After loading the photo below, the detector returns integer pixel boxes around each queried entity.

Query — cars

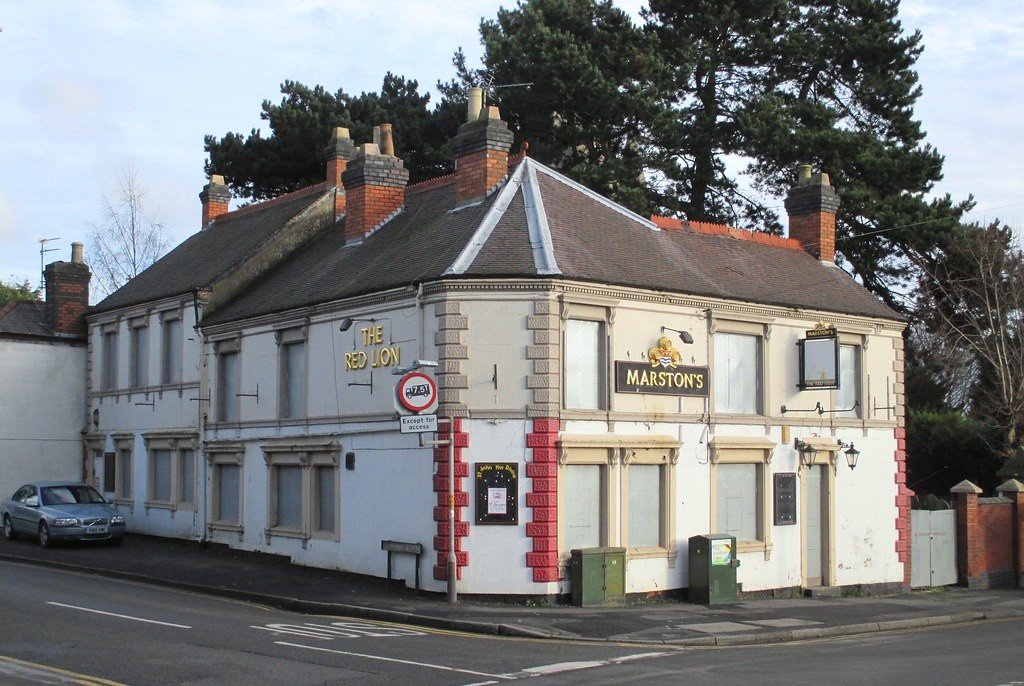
[0,479,127,549]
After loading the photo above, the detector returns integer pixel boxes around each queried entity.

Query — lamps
[838,439,860,471]
[795,438,817,470]
[662,326,694,344]
[340,317,375,332]
